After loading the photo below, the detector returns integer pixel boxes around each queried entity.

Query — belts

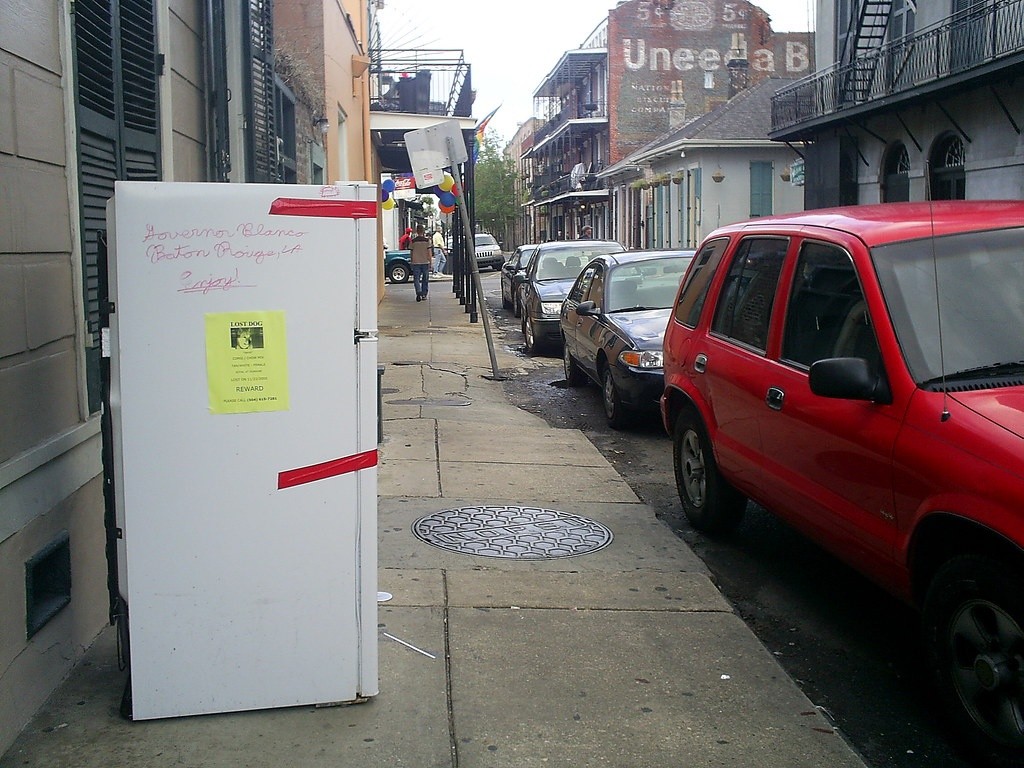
[434,247,440,248]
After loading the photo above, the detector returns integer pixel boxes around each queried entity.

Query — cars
[383,243,412,284]
[514,241,629,351]
[500,244,540,317]
[559,250,698,428]
[460,233,505,270]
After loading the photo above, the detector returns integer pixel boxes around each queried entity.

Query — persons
[399,228,412,250]
[433,226,447,278]
[579,225,594,239]
[411,224,432,302]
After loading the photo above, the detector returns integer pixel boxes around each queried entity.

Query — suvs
[660,198,1024,768]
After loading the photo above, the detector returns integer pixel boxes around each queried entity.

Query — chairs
[539,256,558,277]
[566,255,582,276]
[610,280,641,309]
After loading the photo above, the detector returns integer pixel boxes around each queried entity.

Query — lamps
[312,117,330,134]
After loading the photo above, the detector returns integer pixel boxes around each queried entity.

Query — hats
[435,226,442,231]
[405,228,412,232]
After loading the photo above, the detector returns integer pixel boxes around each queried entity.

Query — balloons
[382,190,389,202]
[382,198,394,210]
[434,175,459,213]
[383,179,395,192]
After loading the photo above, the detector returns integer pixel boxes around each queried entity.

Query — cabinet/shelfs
[399,70,432,115]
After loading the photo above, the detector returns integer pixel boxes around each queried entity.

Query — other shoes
[433,273,438,278]
[422,294,426,300]
[416,296,421,302]
[438,272,444,276]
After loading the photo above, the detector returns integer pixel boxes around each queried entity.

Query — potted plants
[712,171,725,182]
[779,169,790,181]
[630,171,684,191]
